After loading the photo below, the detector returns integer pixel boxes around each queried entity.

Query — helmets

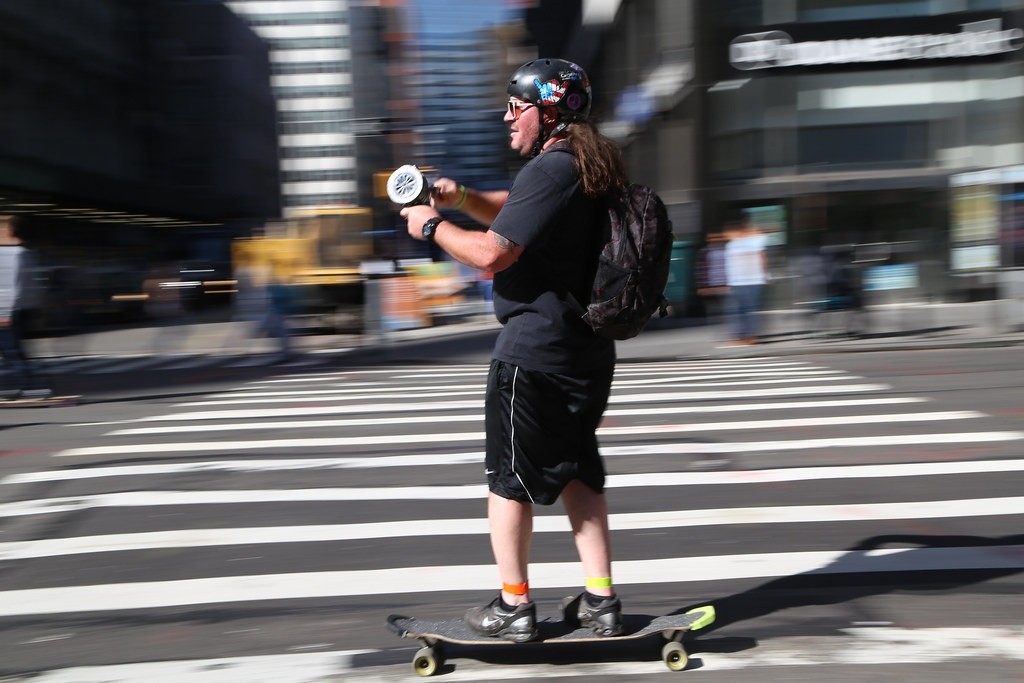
[506,58,592,124]
[548,121,548,124]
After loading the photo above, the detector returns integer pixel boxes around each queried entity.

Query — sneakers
[465,589,539,643]
[559,591,625,637]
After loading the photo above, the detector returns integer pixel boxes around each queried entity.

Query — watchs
[422,216,446,241]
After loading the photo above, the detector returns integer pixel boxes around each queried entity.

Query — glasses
[507,101,534,119]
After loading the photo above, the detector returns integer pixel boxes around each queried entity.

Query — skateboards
[382,604,716,678]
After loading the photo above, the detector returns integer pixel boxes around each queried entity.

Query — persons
[400,58,624,643]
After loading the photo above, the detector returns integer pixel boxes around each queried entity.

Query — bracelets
[452,184,467,210]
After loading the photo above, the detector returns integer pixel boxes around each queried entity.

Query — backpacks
[540,140,673,341]
